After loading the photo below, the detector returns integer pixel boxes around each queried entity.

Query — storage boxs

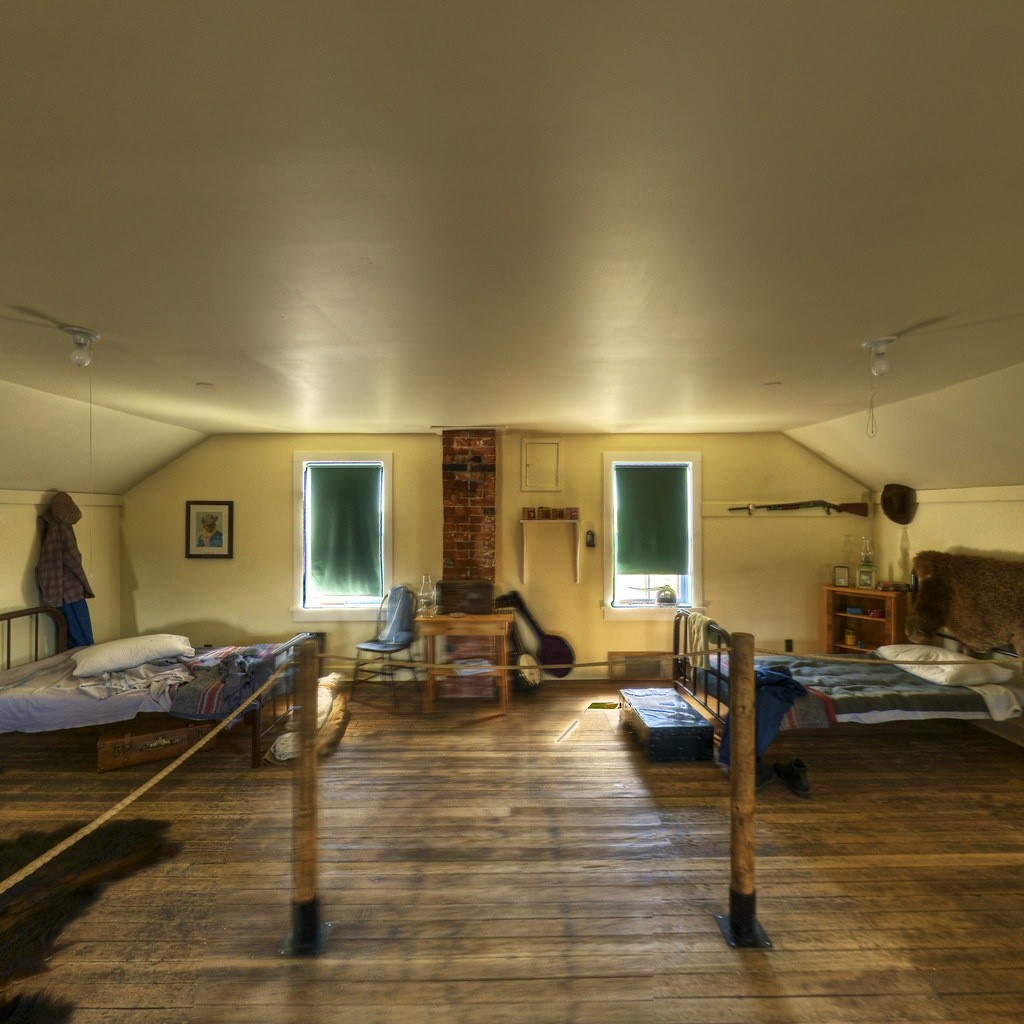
[436,581,497,614]
[619,687,714,759]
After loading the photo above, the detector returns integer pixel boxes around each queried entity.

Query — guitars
[502,592,578,688]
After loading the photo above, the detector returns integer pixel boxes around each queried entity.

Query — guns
[728,498,868,516]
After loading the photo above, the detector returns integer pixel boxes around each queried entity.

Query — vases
[859,535,878,564]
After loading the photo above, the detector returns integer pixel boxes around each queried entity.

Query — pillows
[71,632,195,678]
[877,644,1014,686]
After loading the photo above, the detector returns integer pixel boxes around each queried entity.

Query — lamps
[855,568,875,588]
[862,337,896,376]
[62,323,103,367]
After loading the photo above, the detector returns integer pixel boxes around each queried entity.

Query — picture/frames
[418,573,434,618]
[521,437,565,492]
[834,564,848,587]
[185,500,233,559]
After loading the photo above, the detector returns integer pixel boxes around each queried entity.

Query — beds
[0,607,320,769]
[671,550,1023,763]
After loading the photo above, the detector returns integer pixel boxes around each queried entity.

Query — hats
[201,514,219,524]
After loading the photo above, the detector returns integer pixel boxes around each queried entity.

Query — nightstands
[824,582,908,653]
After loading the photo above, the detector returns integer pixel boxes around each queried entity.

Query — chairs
[349,584,417,709]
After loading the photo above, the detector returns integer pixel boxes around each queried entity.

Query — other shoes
[773,759,810,797]
[756,756,776,793]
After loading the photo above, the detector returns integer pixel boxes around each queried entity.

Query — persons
[197,514,222,547]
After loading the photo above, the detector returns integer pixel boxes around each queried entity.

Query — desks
[421,611,514,716]
